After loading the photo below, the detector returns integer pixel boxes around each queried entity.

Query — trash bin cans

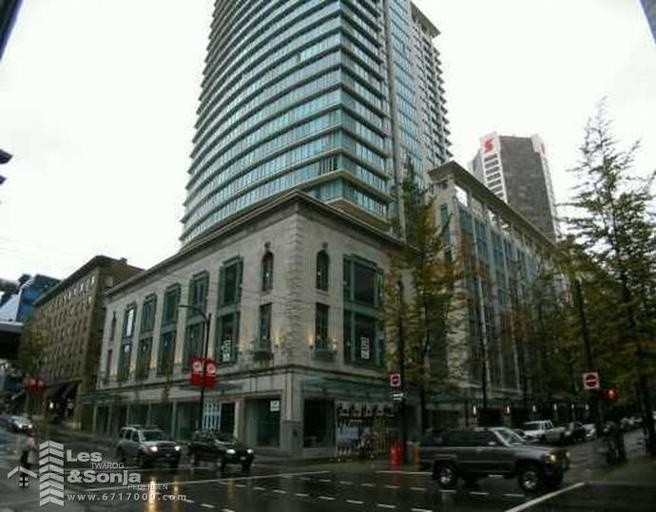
[596,441,614,469]
[391,442,401,465]
[407,441,420,463]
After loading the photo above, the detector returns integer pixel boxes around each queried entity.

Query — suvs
[114,423,182,470]
[417,425,572,495]
[187,428,256,471]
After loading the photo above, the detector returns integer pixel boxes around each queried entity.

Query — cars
[7,415,33,434]
[511,408,656,444]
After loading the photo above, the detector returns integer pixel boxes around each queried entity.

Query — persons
[20,437,37,489]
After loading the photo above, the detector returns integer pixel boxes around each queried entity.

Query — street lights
[175,303,212,429]
[463,398,590,425]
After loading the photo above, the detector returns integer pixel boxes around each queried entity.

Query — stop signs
[390,373,401,387]
[583,373,600,389]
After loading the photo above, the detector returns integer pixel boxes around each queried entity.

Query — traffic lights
[607,386,618,405]
